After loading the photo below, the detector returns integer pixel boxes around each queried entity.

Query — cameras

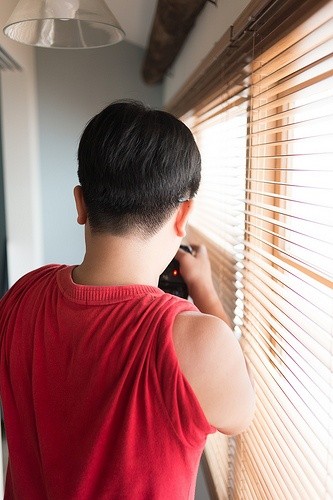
[159,245,191,300]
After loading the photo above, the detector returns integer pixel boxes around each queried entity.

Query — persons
[0,97,254,500]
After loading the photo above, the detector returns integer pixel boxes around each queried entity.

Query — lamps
[3,0,126,50]
